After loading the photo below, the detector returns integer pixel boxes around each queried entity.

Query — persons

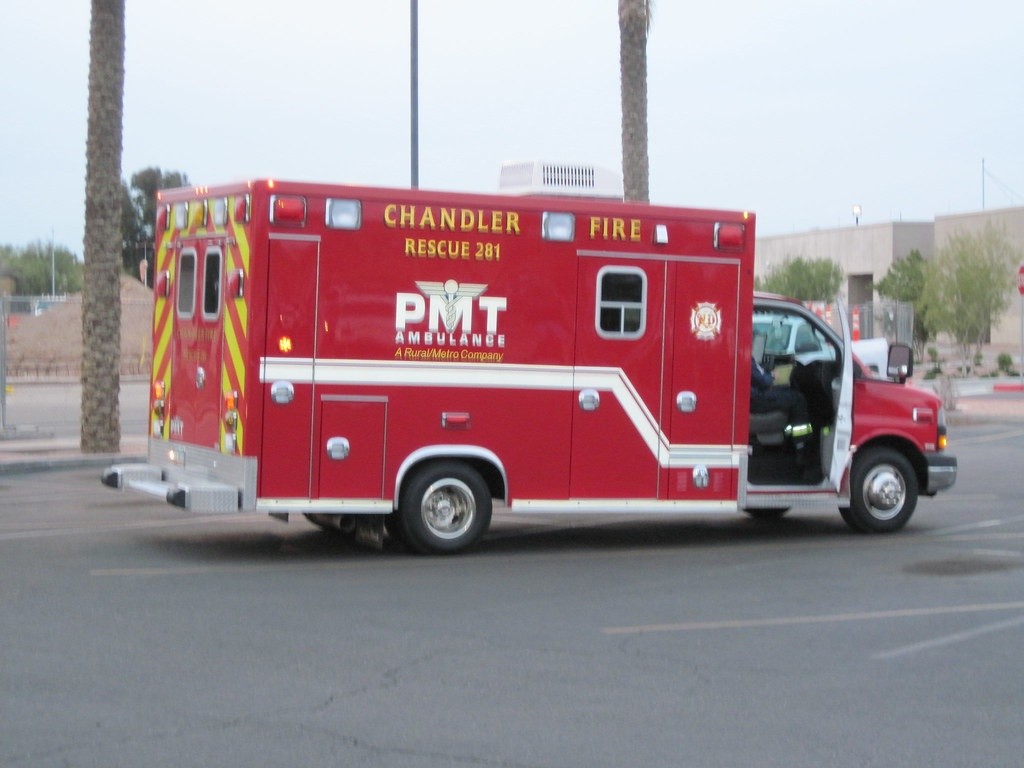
[750,355,815,466]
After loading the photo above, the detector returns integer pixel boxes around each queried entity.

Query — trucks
[752,312,898,387]
[99,158,958,560]
